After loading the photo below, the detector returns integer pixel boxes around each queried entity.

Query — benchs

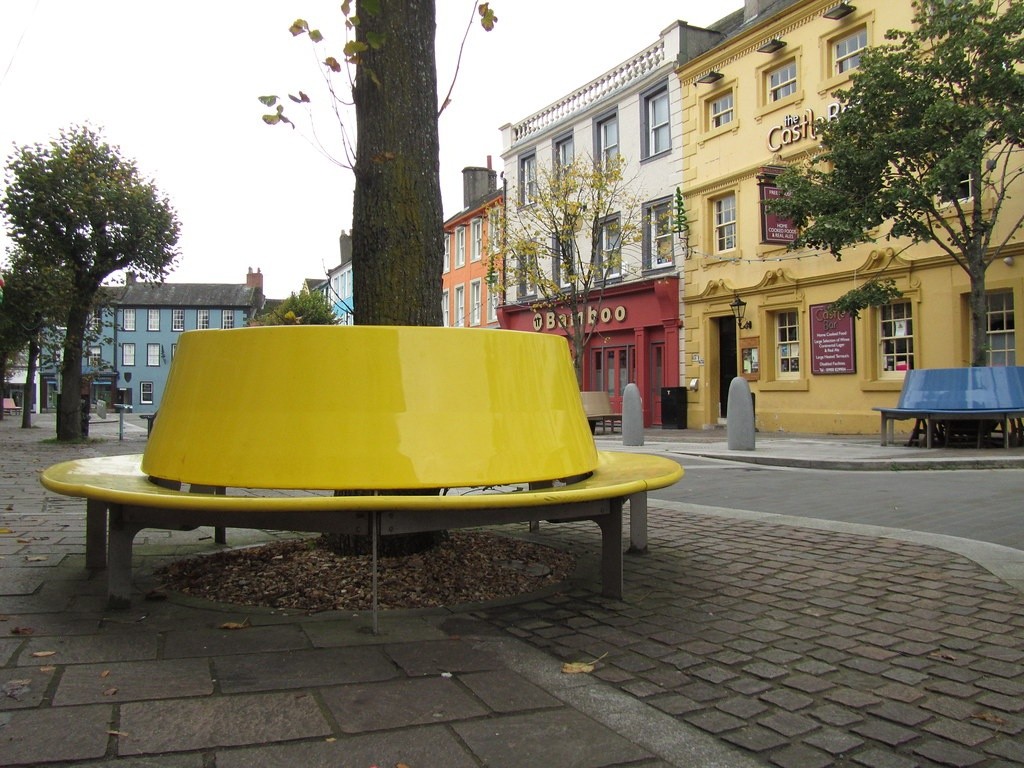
[41,454,686,602]
[3,398,22,416]
[872,366,1024,449]
[580,391,622,434]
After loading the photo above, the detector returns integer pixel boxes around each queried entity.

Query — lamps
[823,3,856,21]
[697,71,724,83]
[729,295,752,330]
[757,38,787,53]
